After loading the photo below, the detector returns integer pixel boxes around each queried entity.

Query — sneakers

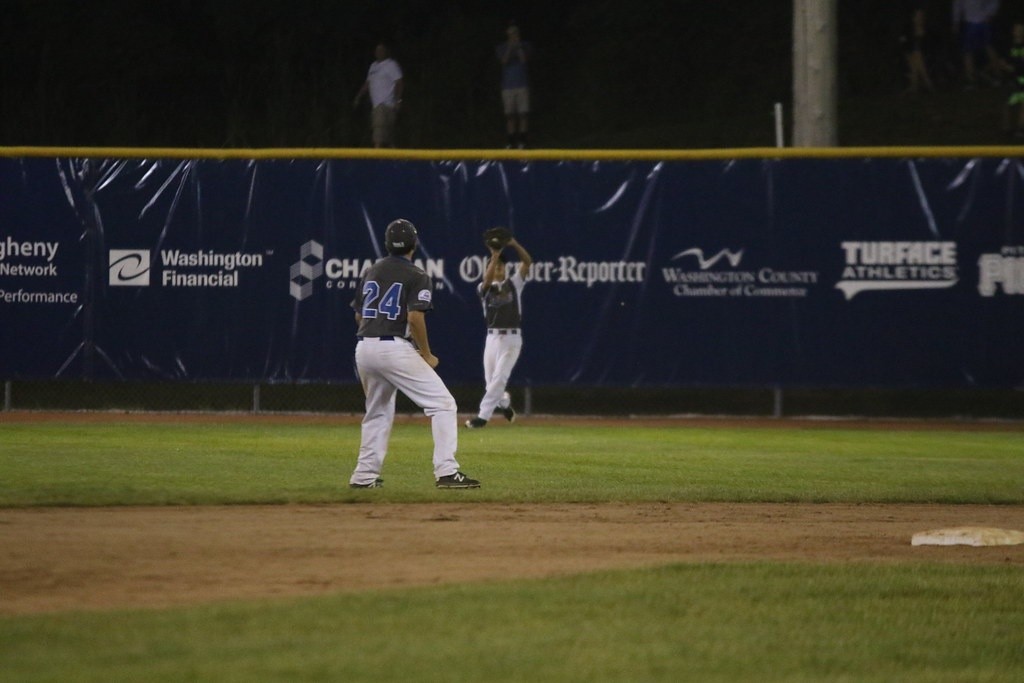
[436,472,480,489]
[349,478,384,489]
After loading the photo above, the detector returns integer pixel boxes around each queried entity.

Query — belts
[357,336,394,341]
[488,330,517,334]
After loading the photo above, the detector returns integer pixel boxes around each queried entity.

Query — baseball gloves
[484,226,512,250]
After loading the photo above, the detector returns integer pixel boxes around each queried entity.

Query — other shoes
[503,405,515,423]
[465,418,486,428]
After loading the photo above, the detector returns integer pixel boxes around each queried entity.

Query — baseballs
[491,237,499,242]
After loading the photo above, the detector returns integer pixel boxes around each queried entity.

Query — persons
[353,44,405,149]
[349,219,481,489]
[496,25,529,149]
[901,0,1024,137]
[465,227,532,429]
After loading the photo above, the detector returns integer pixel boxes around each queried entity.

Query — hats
[385,219,417,248]
[486,254,508,267]
